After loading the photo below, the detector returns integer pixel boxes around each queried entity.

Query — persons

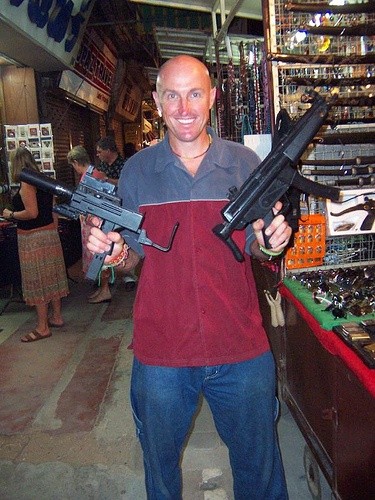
[67,144,115,304]
[93,136,126,189]
[123,142,138,158]
[88,54,292,500]
[3,147,71,343]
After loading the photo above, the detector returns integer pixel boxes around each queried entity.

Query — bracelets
[105,175,109,182]
[10,212,15,219]
[258,243,284,256]
[98,244,130,287]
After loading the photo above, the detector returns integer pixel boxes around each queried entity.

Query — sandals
[88,289,112,304]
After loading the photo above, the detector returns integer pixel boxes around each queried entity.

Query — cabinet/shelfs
[251,0,375,500]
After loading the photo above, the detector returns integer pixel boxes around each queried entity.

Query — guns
[211,89,341,263]
[18,164,180,283]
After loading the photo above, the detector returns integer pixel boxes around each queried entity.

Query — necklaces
[168,132,212,160]
[214,40,271,143]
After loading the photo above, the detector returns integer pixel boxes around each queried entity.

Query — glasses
[96,150,107,154]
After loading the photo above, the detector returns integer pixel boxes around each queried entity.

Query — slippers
[46,319,63,327]
[20,329,52,342]
